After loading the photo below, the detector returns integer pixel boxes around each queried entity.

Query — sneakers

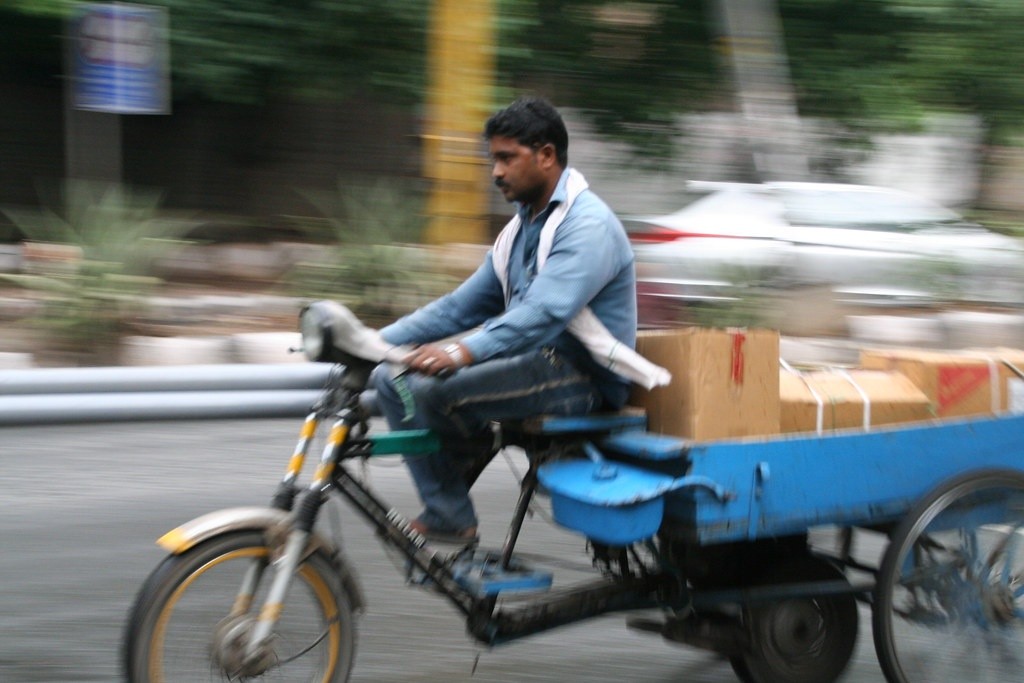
[406,517,479,544]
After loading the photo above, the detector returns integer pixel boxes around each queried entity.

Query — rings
[421,357,435,368]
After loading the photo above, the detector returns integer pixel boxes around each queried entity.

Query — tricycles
[120,299,1024,683]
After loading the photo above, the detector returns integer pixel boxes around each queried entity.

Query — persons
[373,95,640,546]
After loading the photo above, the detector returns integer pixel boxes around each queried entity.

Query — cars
[620,179,1024,349]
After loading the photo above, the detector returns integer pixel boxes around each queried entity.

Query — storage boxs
[856,345,1024,422]
[628,325,782,439]
[776,368,932,433]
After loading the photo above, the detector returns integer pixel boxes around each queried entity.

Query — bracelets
[442,343,466,367]
